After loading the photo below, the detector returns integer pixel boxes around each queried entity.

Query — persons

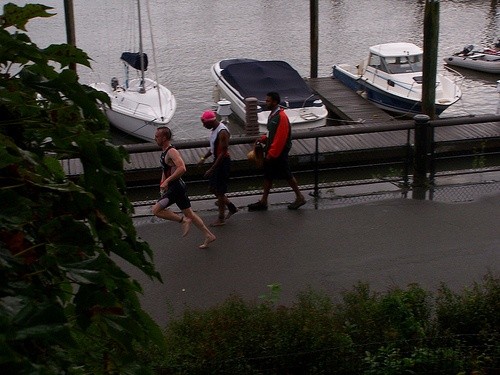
[248,91,307,210]
[152,127,216,249]
[196,110,240,227]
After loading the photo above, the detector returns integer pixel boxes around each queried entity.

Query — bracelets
[200,157,205,161]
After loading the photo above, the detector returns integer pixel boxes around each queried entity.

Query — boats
[210,58,328,134]
[443,45,500,74]
[87,0,177,143]
[330,42,465,117]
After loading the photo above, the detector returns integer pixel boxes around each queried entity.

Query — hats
[201,111,216,121]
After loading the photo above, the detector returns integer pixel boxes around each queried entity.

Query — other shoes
[288,200,306,209]
[249,202,267,211]
